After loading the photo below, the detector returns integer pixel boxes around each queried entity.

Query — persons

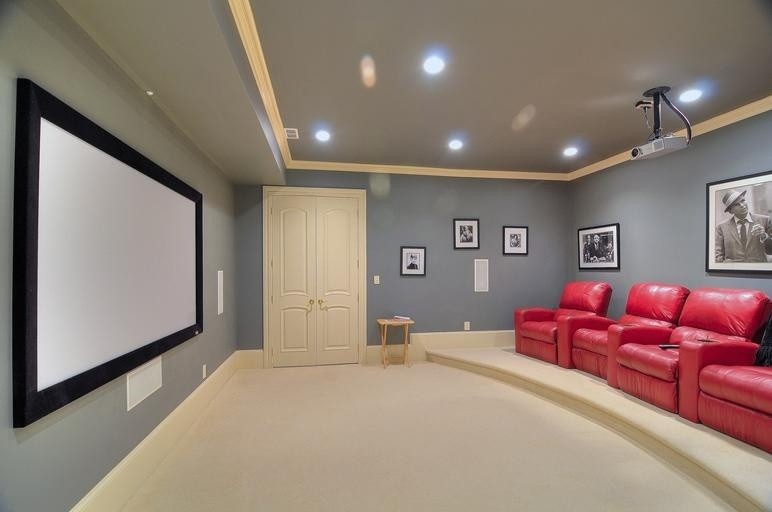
[460,225,473,242]
[715,189,772,263]
[406,253,419,269]
[511,235,519,248]
[583,234,613,262]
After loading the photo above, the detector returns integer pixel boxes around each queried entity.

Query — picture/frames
[453,218,480,249]
[578,223,620,270]
[400,245,426,276]
[503,226,528,255]
[706,169,772,275]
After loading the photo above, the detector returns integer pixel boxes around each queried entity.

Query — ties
[739,220,747,245]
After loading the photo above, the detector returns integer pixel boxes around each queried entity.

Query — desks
[377,319,415,369]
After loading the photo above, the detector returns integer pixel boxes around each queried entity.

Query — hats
[723,189,746,212]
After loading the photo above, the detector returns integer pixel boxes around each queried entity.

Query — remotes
[659,345,680,349]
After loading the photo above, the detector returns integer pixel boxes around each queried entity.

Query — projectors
[630,136,688,160]
